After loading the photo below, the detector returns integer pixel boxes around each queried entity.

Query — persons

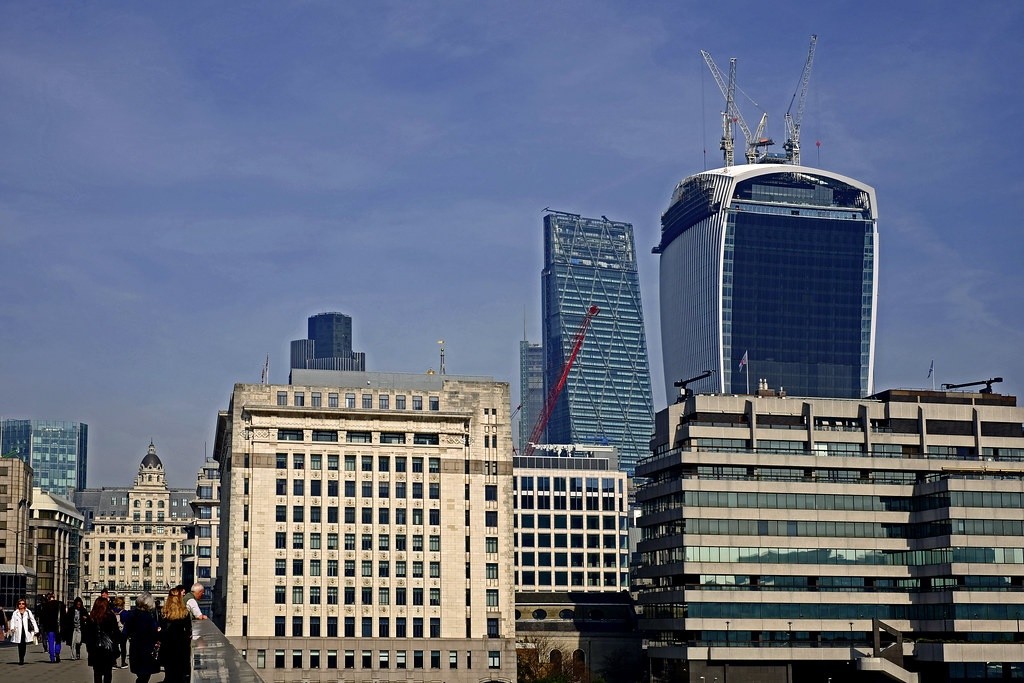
[83,597,122,683]
[66,597,90,660]
[101,588,128,670]
[124,591,161,683]
[157,583,207,683]
[36,592,66,663]
[10,599,39,665]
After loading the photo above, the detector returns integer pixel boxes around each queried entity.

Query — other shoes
[19,661,24,664]
[121,662,128,669]
[51,659,56,663]
[56,653,60,663]
[112,665,118,670]
[76,654,80,659]
[70,655,75,659]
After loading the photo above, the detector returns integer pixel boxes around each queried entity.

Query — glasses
[18,603,25,605]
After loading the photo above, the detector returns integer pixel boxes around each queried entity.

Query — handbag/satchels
[27,611,34,632]
[151,641,161,660]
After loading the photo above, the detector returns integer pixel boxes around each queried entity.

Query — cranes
[511,304,600,456]
[700,34,818,171]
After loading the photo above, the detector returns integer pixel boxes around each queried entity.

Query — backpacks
[112,609,125,632]
[94,616,119,653]
